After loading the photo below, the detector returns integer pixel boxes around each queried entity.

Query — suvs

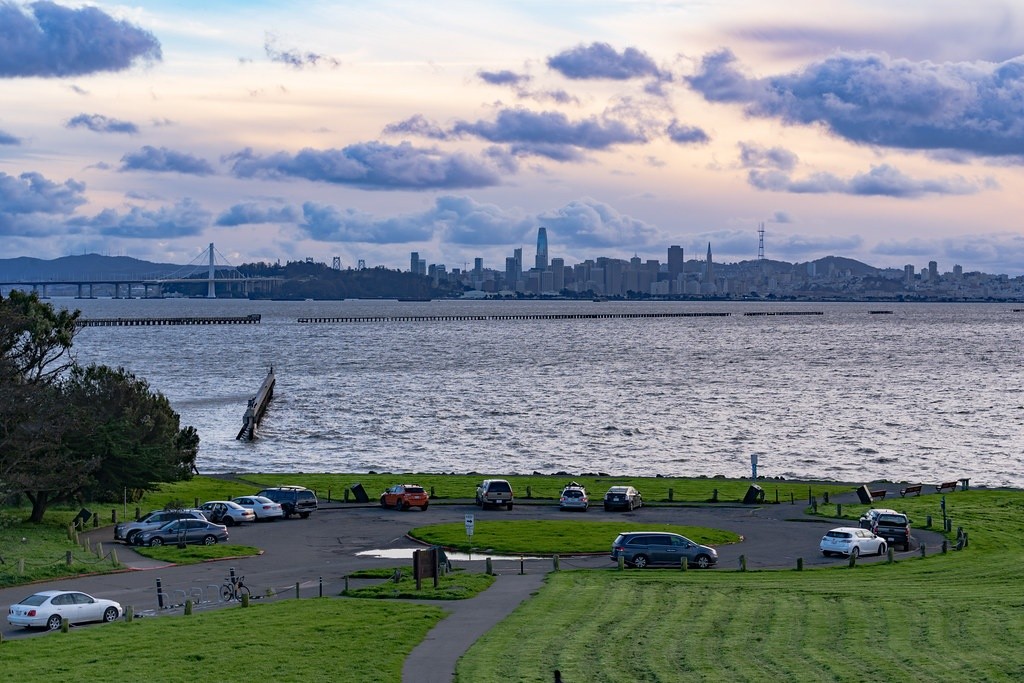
[558,485,591,512]
[256,486,318,519]
[475,480,515,510]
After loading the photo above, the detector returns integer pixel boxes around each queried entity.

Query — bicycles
[220,576,252,603]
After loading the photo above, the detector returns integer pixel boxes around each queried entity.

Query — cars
[6,589,123,630]
[232,496,285,522]
[112,509,229,548]
[611,532,719,568]
[821,527,886,558]
[378,484,431,513]
[605,486,644,512]
[197,501,256,525]
[859,507,914,549]
